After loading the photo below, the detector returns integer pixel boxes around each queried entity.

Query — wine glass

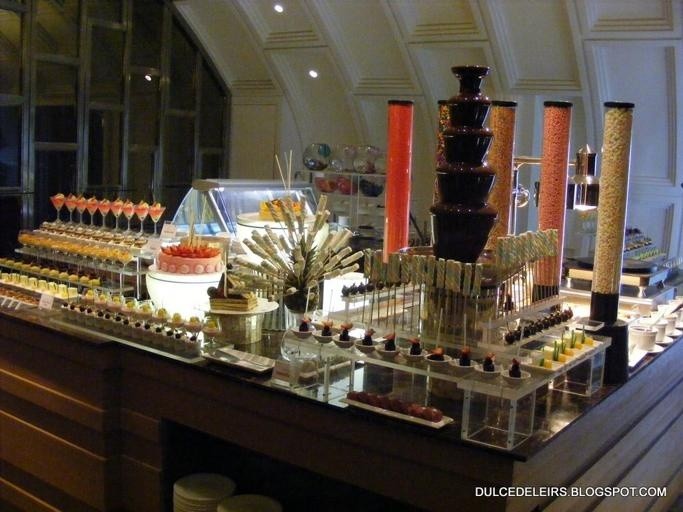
[64,200,77,228]
[121,207,136,234]
[148,206,167,237]
[50,197,66,224]
[97,205,109,230]
[75,201,87,231]
[110,206,122,236]
[85,202,99,228]
[134,205,149,235]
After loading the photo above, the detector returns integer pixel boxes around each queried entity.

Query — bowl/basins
[354,338,378,354]
[453,359,479,375]
[424,353,452,368]
[400,348,428,362]
[629,325,658,352]
[314,176,385,197]
[502,369,532,384]
[374,344,401,358]
[477,363,501,380]
[637,318,670,342]
[331,335,357,348]
[292,326,314,338]
[311,330,336,343]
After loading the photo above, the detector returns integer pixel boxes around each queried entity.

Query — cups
[80,288,223,340]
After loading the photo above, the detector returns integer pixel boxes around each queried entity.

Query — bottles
[301,143,379,174]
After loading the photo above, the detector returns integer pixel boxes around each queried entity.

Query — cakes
[155,243,222,275]
[258,199,300,221]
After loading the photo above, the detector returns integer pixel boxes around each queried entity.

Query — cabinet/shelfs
[293,170,386,237]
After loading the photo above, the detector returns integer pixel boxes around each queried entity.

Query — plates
[521,333,600,372]
[656,336,674,345]
[338,397,454,429]
[201,346,276,373]
[171,472,283,512]
[647,344,664,354]
[670,329,682,337]
